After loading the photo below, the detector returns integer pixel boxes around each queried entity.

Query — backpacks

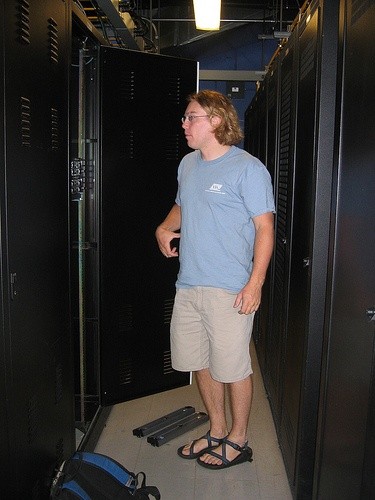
[51,451,162,500]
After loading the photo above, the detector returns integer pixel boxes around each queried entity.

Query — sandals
[196,434,253,470]
[178,428,232,459]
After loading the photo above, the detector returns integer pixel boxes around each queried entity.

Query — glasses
[181,114,212,124]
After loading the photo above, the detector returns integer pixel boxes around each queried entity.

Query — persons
[154,90,277,469]
